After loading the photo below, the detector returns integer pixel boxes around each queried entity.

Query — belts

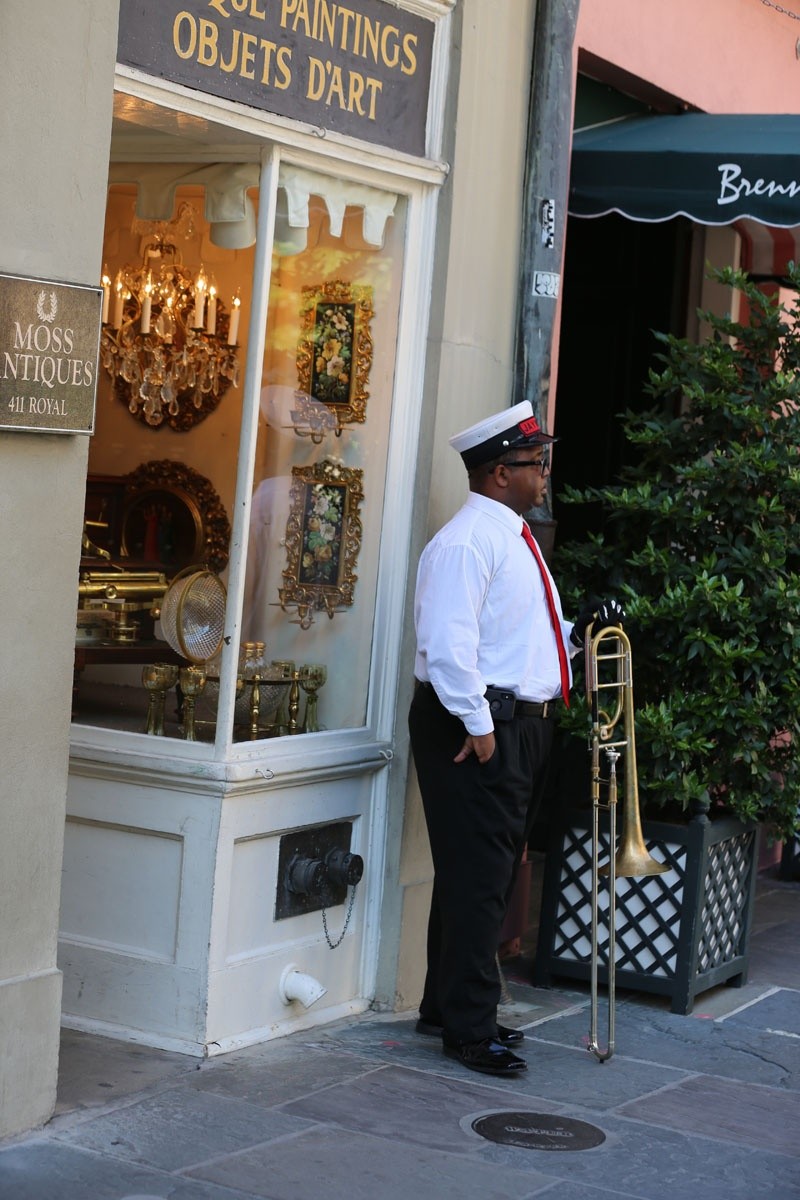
[516,699,558,718]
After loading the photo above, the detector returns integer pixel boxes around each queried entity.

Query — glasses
[488,457,548,472]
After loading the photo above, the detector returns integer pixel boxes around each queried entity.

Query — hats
[448,400,556,471]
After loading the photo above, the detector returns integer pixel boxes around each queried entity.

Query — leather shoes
[441,1036,529,1072]
[416,1013,525,1044]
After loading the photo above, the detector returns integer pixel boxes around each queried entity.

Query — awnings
[570,114,800,230]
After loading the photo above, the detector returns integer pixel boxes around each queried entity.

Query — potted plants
[532,258,799,1016]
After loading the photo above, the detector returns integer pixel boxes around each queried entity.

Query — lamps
[101,203,243,431]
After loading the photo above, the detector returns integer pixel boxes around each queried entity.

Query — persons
[406,399,574,1075]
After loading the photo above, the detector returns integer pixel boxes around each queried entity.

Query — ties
[521,522,571,710]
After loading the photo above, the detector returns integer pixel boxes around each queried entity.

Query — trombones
[581,620,674,1066]
